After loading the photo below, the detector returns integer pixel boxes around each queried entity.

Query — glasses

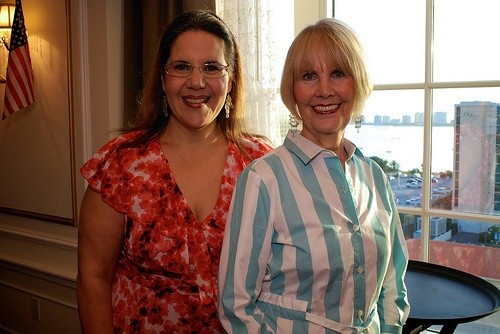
[163,60,230,77]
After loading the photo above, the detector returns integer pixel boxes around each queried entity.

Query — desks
[402,259,500,334]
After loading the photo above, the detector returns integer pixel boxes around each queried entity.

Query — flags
[2,0,38,121]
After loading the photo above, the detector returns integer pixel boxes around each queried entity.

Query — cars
[386,169,451,207]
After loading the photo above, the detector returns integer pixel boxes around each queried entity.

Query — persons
[218,18,410,334]
[78,10,273,334]
